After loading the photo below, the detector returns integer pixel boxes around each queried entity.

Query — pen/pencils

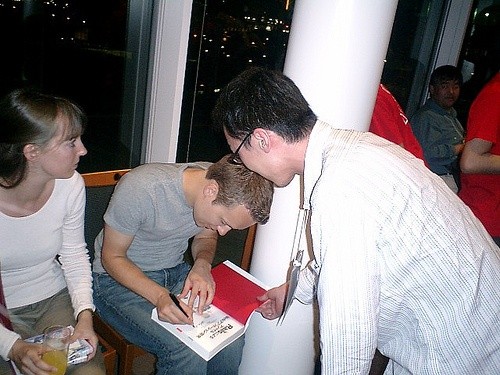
[169,293,194,327]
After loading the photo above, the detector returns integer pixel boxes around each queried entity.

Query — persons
[92,155,273,375]
[211,67,499,374]
[411,65,466,193]
[0,91,106,374]
[456,72,500,245]
[369,84,428,167]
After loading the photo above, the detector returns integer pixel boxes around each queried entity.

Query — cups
[41,324,71,375]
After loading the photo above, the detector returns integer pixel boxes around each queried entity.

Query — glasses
[227,132,253,165]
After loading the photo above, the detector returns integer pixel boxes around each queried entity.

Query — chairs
[0,169,256,375]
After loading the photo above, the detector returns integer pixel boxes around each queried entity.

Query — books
[9,325,93,375]
[150,259,273,362]
